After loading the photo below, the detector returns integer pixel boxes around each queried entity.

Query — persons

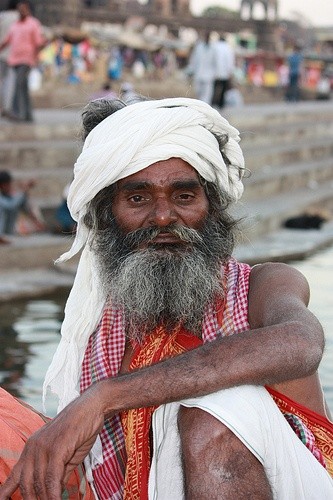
[0,0,332,123]
[0,98,333,500]
[0,171,36,244]
